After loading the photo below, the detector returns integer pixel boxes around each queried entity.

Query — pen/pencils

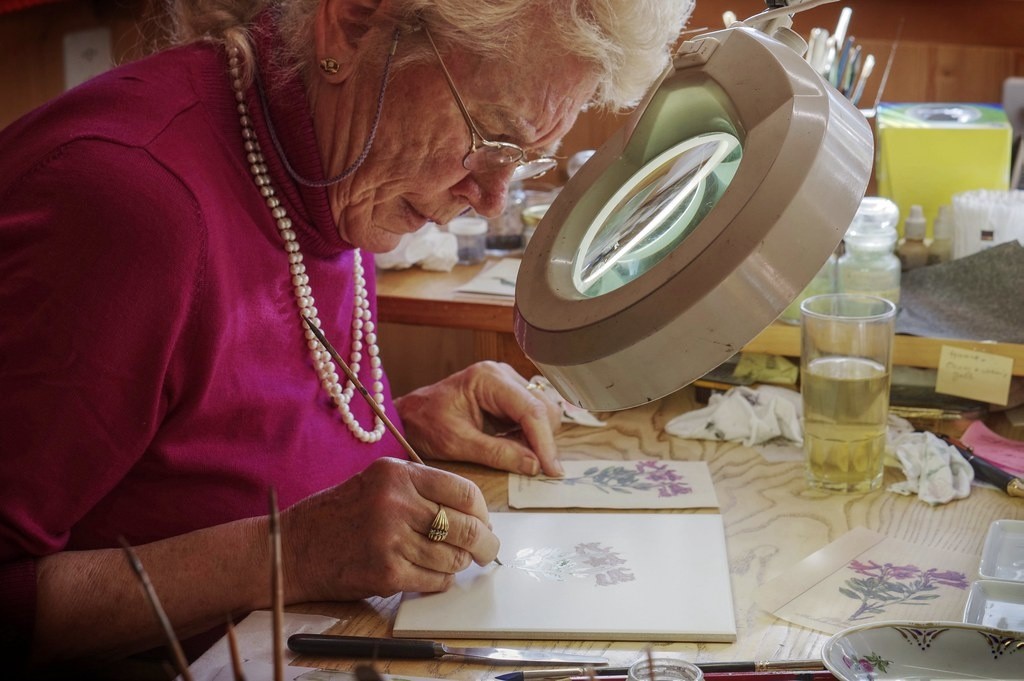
[914,428,1024,500]
[807,7,877,106]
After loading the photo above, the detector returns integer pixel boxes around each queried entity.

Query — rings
[429,503,449,541]
[524,380,546,393]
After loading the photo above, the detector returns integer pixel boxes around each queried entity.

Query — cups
[626,657,704,681]
[951,190,1024,263]
[799,293,896,496]
[446,218,488,265]
[488,182,526,255]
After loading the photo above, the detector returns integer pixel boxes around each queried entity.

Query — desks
[170,254,1024,681]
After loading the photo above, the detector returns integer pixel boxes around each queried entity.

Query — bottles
[897,206,953,271]
[779,197,902,325]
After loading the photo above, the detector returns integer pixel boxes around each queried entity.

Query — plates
[979,518,1024,582]
[960,580,1024,633]
[820,621,1024,681]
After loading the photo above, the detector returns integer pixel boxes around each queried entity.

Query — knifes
[287,632,610,664]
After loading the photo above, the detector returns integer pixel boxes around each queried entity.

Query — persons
[0,0,699,681]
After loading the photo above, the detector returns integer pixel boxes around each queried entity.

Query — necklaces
[229,40,389,443]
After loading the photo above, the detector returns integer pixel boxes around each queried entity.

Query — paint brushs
[493,644,841,681]
[115,487,286,681]
[301,311,503,566]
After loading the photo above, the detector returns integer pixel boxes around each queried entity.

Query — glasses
[419,14,557,182]
